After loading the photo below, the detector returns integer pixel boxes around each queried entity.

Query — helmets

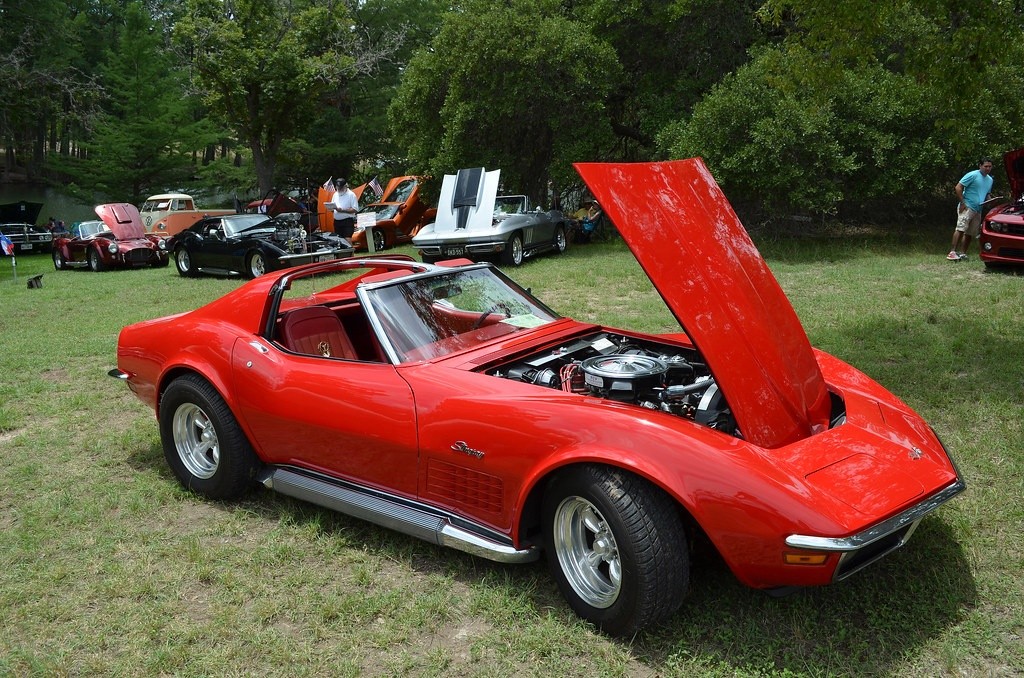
[48,221,55,227]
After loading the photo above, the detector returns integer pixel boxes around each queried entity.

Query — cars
[107,156,968,637]
[411,167,567,268]
[67,221,82,238]
[245,198,318,232]
[979,147,1024,275]
[165,213,357,280]
[0,200,53,256]
[41,224,72,250]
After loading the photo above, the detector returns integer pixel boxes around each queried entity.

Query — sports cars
[318,175,438,253]
[51,202,170,271]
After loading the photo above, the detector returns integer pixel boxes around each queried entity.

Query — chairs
[565,209,605,244]
[283,306,359,361]
[368,295,443,363]
[207,225,220,236]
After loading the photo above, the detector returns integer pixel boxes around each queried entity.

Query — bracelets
[960,201,966,205]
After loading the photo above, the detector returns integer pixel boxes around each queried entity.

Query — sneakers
[946,252,961,260]
[959,253,969,260]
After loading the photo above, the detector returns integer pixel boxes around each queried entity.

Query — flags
[323,179,335,192]
[368,177,383,196]
[1,232,15,256]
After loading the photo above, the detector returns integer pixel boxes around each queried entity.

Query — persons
[330,178,359,248]
[44,217,69,239]
[947,158,993,260]
[571,200,600,232]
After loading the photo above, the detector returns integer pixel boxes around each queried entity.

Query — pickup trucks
[139,194,238,234]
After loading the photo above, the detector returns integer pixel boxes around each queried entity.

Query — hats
[335,178,346,186]
[593,198,600,207]
[584,197,592,202]
[58,220,64,224]
